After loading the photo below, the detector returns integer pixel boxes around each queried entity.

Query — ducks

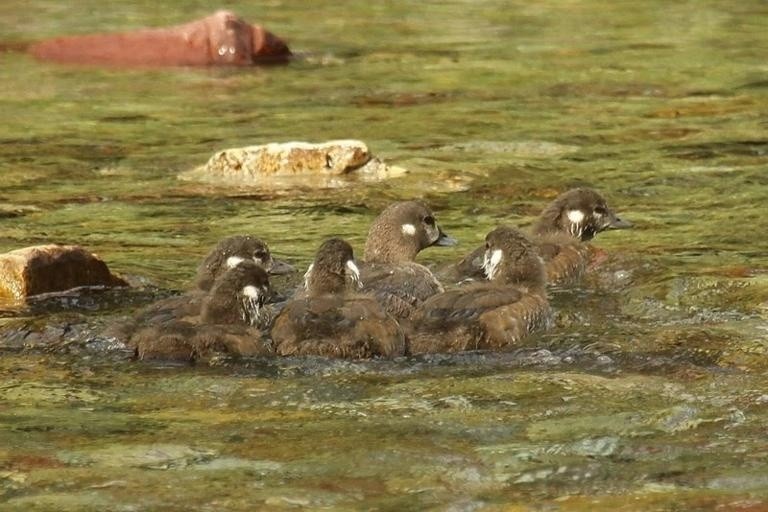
[100,188,633,370]
